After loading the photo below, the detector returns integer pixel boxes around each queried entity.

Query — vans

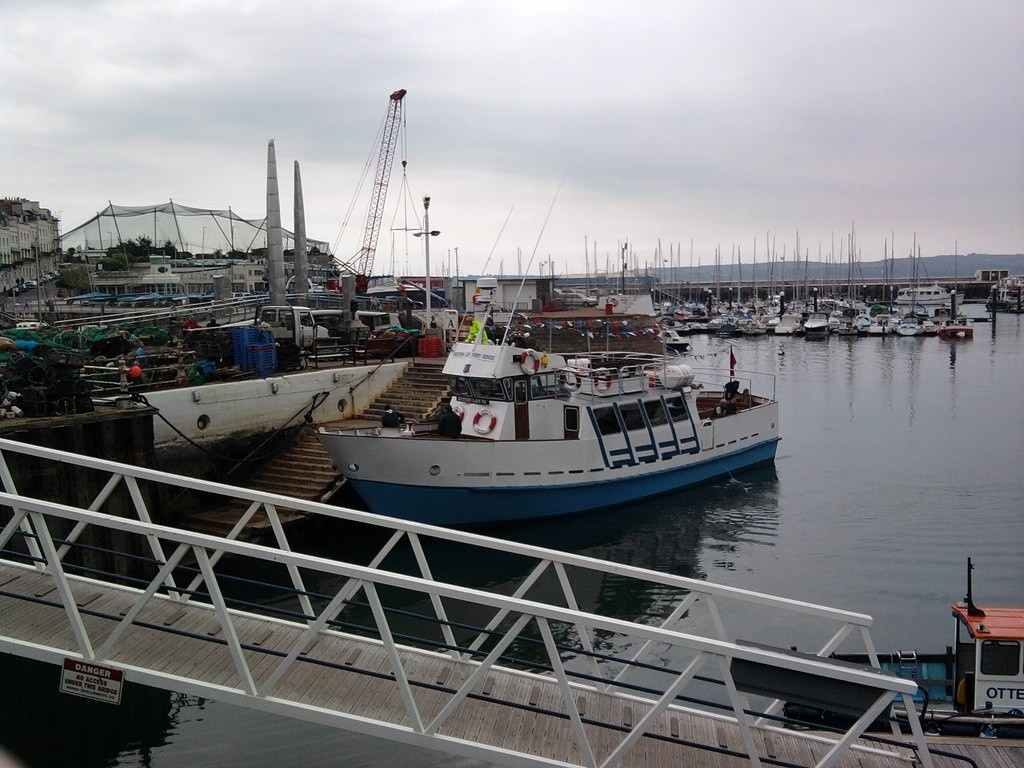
[310,310,390,335]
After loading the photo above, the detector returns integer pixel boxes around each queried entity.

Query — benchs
[302,322,373,369]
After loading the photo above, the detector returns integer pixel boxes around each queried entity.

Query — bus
[59,263,71,272]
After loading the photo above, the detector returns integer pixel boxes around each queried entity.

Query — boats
[897,283,965,305]
[985,275,1024,313]
[781,557,1024,738]
[315,179,783,527]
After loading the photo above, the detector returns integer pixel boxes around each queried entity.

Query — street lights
[412,196,441,328]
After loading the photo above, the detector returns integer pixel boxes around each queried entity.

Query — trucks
[183,304,318,356]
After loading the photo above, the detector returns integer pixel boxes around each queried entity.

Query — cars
[388,298,423,309]
[171,260,194,268]
[218,261,228,266]
[194,261,205,267]
[18,284,28,292]
[26,281,37,289]
[37,271,58,285]
[552,293,599,307]
[8,287,21,296]
[228,260,254,265]
[205,261,216,266]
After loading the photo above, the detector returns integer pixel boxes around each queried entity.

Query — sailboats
[865,231,898,334]
[896,232,935,336]
[586,236,706,350]
[936,242,974,335]
[707,226,866,335]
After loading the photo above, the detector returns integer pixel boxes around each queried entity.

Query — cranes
[325,88,408,302]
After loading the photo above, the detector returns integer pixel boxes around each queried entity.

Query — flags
[730,351,737,379]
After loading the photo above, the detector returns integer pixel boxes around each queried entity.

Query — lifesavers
[521,349,541,375]
[450,405,464,423]
[561,368,581,392]
[473,408,496,433]
[594,367,613,392]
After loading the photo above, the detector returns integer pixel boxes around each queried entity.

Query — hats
[466,317,473,321]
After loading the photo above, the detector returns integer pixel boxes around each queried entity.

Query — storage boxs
[229,328,277,375]
[420,337,442,358]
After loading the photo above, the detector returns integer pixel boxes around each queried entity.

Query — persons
[464,316,488,345]
[381,404,405,427]
[3,285,6,291]
[16,277,24,286]
[438,404,462,439]
[23,297,29,310]
